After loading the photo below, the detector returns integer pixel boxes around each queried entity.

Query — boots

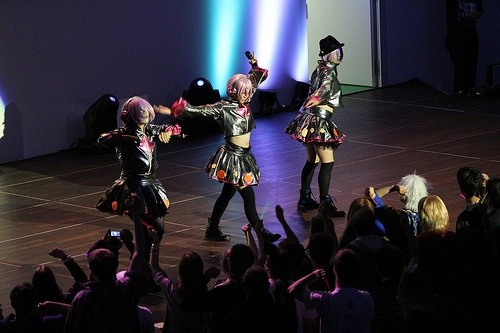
[297,188,319,211]
[255,222,279,245]
[204,218,230,240]
[319,195,346,217]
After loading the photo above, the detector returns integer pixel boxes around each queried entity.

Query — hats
[318,35,345,57]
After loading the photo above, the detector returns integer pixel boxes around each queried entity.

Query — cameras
[108,230,121,237]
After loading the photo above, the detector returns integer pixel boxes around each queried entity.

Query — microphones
[245,51,253,60]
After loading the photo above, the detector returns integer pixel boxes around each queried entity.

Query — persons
[283,35,346,217]
[0,165,499,333]
[94,95,188,291]
[153,49,281,249]
